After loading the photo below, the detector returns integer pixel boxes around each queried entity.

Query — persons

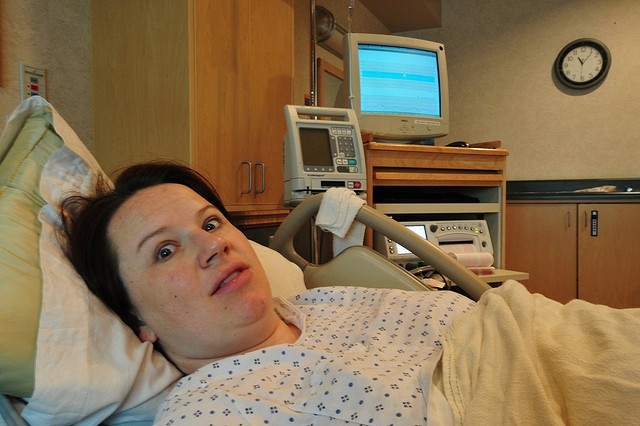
[58,162,478,426]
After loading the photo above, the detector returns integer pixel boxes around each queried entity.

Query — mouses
[446,141,470,147]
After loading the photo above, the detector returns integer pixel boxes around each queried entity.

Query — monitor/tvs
[344,32,449,144]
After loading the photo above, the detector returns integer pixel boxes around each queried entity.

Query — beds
[0,191,492,426]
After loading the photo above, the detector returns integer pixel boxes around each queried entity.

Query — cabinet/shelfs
[93,0,294,213]
[506,199,639,310]
[359,132,531,292]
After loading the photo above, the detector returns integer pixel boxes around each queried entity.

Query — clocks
[553,36,612,90]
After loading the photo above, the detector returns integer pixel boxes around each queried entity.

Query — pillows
[0,89,310,402]
